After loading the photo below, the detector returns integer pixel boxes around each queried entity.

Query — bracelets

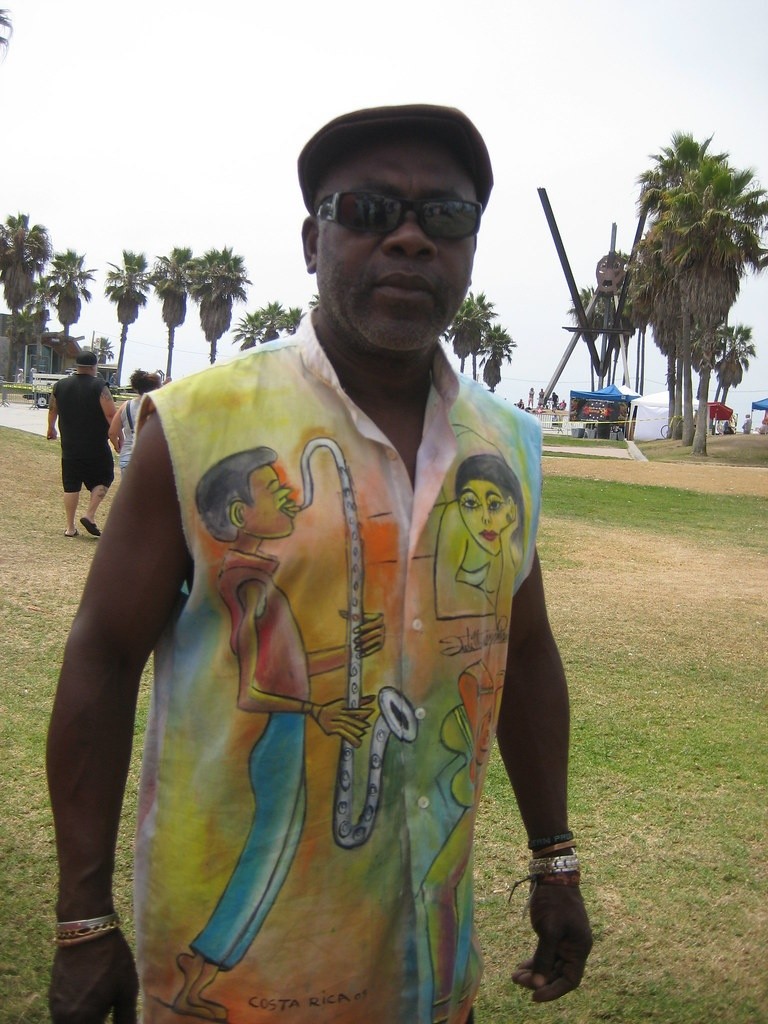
[508,832,581,920]
[53,912,122,946]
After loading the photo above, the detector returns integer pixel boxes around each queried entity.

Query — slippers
[65,529,79,537]
[80,518,101,536]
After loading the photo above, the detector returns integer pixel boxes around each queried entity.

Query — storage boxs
[572,428,585,438]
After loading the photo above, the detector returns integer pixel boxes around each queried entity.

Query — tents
[628,389,702,441]
[751,398,768,435]
[569,384,639,422]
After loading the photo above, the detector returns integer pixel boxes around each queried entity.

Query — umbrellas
[708,402,733,432]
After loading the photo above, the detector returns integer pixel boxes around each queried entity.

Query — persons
[30,365,37,372]
[528,388,534,409]
[539,388,544,398]
[551,391,567,410]
[46,104,592,1024]
[717,418,737,434]
[47,351,125,538]
[17,369,23,383]
[742,414,752,434]
[108,370,162,477]
[516,399,524,409]
[110,373,116,384]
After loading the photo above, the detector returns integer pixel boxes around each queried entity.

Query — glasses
[316,193,482,241]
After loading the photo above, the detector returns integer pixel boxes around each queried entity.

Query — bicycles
[660,415,696,440]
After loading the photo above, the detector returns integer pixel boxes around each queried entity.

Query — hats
[19,369,23,372]
[298,104,494,219]
[73,351,97,367]
